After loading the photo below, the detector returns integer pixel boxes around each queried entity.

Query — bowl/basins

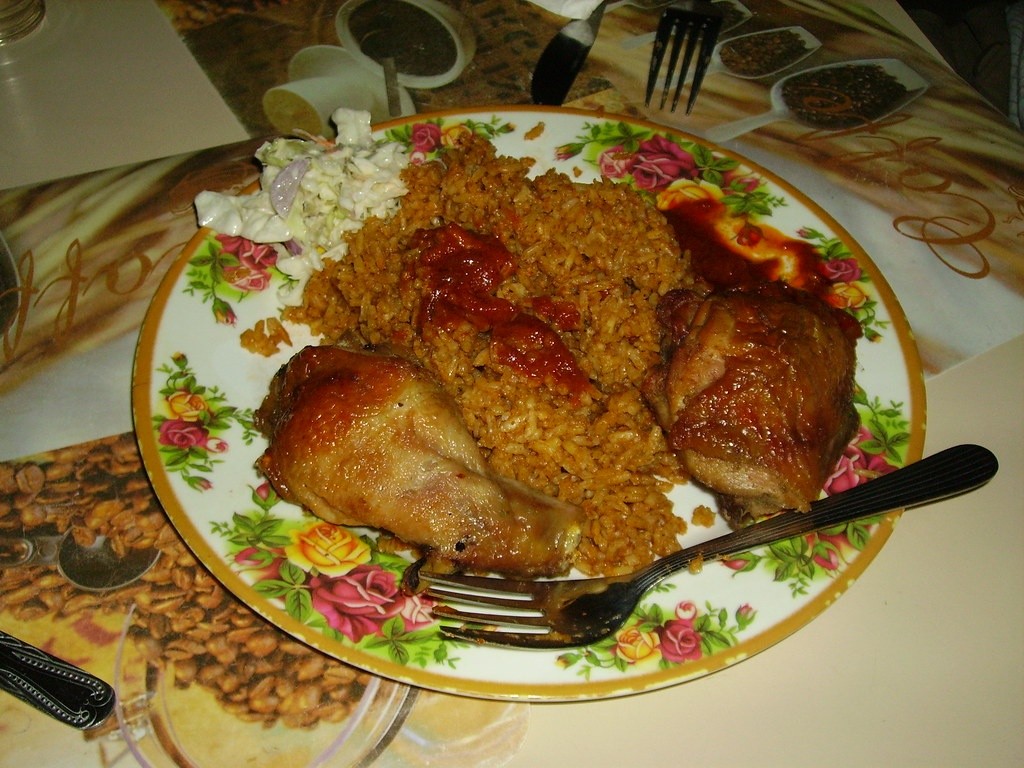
[333,1,479,90]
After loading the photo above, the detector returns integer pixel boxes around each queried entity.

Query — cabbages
[193,108,412,307]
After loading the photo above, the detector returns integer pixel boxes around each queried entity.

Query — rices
[243,123,715,576]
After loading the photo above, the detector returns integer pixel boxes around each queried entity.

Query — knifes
[531,0,607,107]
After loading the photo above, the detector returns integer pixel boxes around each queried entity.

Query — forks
[417,444,999,651]
[644,0,723,115]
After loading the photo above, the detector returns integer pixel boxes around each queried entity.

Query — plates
[288,45,417,122]
[113,590,413,767]
[130,104,927,704]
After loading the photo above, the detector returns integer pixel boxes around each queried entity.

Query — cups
[264,75,379,143]
[0,1,48,46]
